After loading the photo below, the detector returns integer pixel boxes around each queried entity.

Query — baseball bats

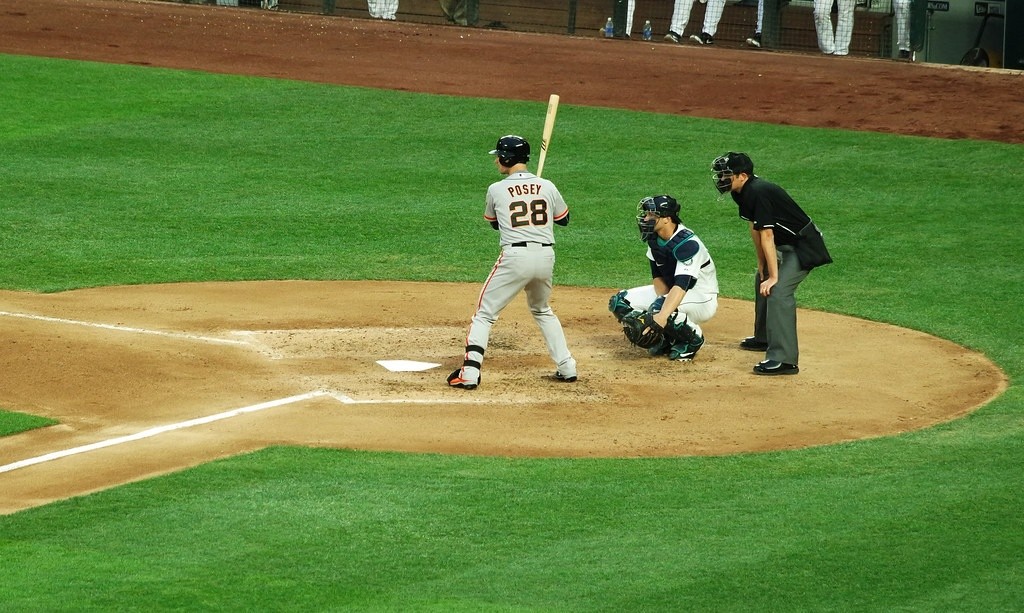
[537,94,560,178]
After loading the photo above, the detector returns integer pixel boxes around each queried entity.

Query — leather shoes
[740,335,769,351]
[753,359,799,375]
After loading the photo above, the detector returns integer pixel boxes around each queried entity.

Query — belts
[512,241,552,247]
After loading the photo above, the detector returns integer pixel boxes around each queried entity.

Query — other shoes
[216,0,238,6]
[669,335,704,362]
[689,32,714,46]
[746,30,771,48]
[451,378,477,389]
[647,336,678,356]
[664,31,681,44]
[553,370,577,382]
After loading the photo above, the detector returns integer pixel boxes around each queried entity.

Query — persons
[187,0,480,25]
[598,1,695,44]
[810,1,857,56]
[710,150,833,377]
[689,0,784,47]
[892,1,912,63]
[609,196,718,361]
[444,133,578,390]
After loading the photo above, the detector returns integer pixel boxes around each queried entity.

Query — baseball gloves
[622,312,664,349]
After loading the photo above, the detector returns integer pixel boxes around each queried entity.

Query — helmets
[713,151,753,175]
[488,135,531,168]
[642,196,682,218]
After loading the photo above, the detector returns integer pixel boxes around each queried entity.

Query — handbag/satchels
[795,221,833,270]
